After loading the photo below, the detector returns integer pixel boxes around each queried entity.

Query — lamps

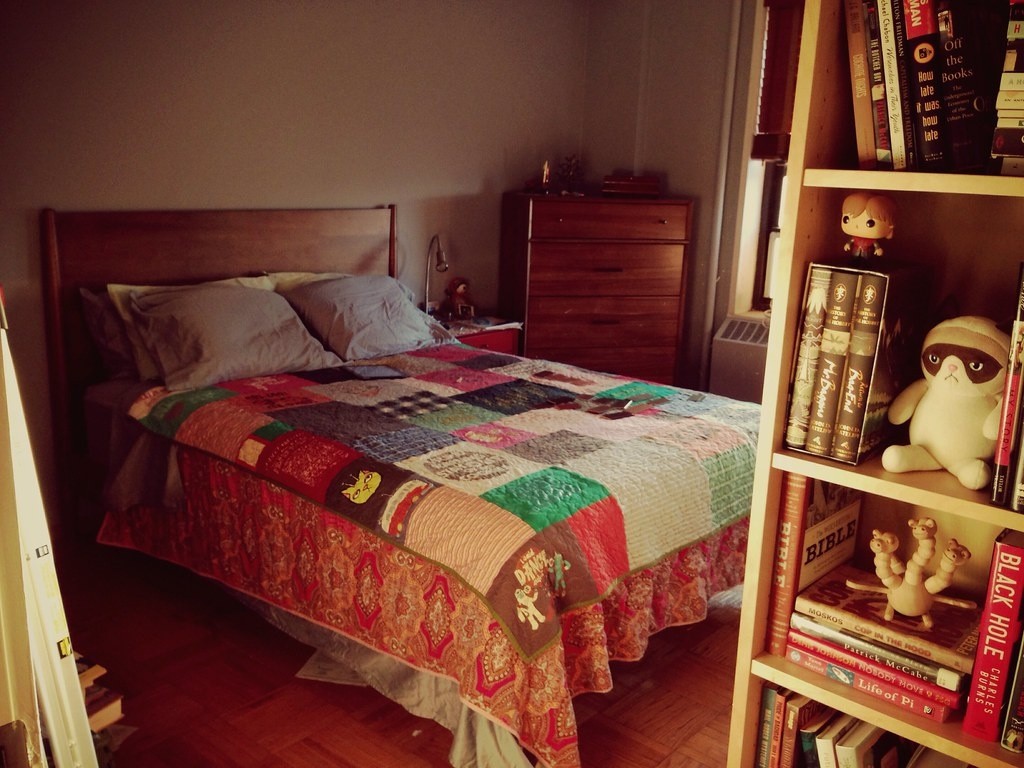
[417,233,449,315]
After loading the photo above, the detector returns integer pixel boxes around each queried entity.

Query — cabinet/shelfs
[497,188,697,391]
[726,0,1023,768]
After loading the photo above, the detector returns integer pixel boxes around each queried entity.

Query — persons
[841,193,895,271]
[444,276,474,320]
[943,15,949,29]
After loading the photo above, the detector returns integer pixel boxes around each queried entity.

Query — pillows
[78,271,463,394]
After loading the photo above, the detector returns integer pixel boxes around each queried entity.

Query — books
[763,470,861,659]
[782,254,933,466]
[785,561,985,723]
[753,680,969,768]
[40,651,125,740]
[961,529,1024,754]
[845,0,1024,170]
[987,260,1024,515]
[459,315,519,329]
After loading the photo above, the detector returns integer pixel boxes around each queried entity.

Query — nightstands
[430,314,520,358]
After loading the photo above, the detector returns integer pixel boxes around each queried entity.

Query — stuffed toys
[881,313,1011,490]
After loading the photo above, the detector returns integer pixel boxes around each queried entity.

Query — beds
[39,197,762,768]
